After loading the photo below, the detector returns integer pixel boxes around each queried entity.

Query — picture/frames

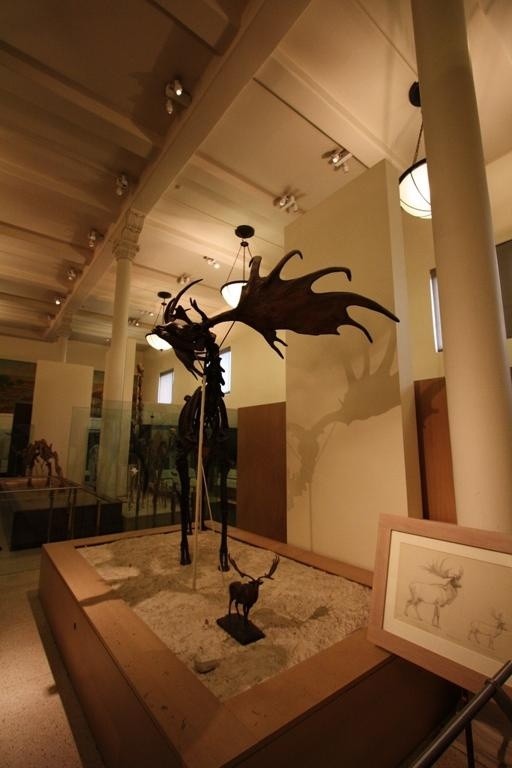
[365,513,512,704]
[0,357,37,416]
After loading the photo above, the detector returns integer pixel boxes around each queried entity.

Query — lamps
[399,82,433,219]
[219,225,255,310]
[143,291,174,351]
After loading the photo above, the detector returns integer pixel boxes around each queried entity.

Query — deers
[467,610,510,651]
[222,552,280,627]
[403,558,464,628]
